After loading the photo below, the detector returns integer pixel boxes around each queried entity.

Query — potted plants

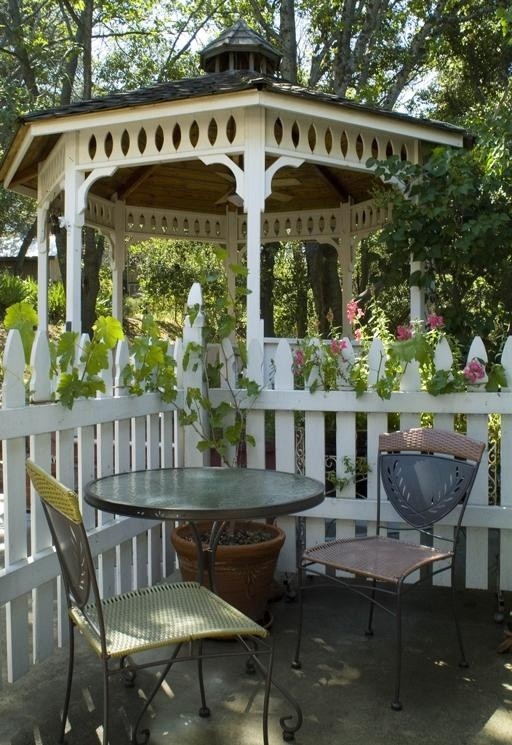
[120,243,286,642]
